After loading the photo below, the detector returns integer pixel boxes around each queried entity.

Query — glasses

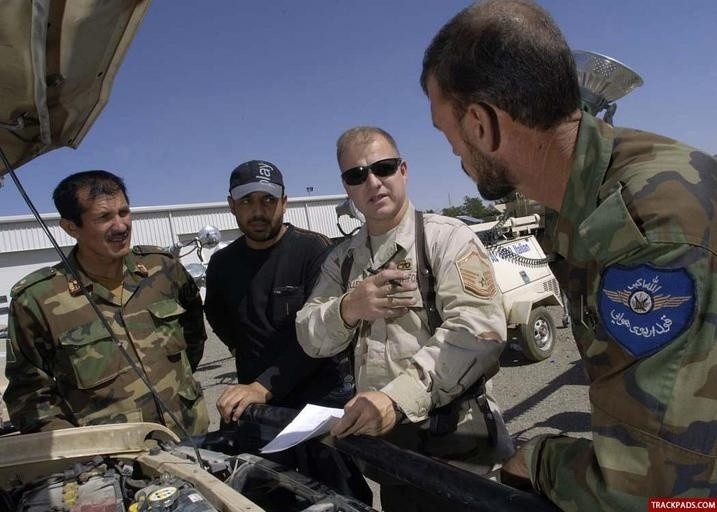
[342,158,402,186]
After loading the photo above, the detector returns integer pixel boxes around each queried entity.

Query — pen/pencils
[367,266,404,288]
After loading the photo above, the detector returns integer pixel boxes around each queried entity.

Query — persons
[420,1,717,512]
[2,170,210,441]
[293,125,516,511]
[203,159,374,508]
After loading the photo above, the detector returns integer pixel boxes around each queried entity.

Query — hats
[230,160,284,203]
